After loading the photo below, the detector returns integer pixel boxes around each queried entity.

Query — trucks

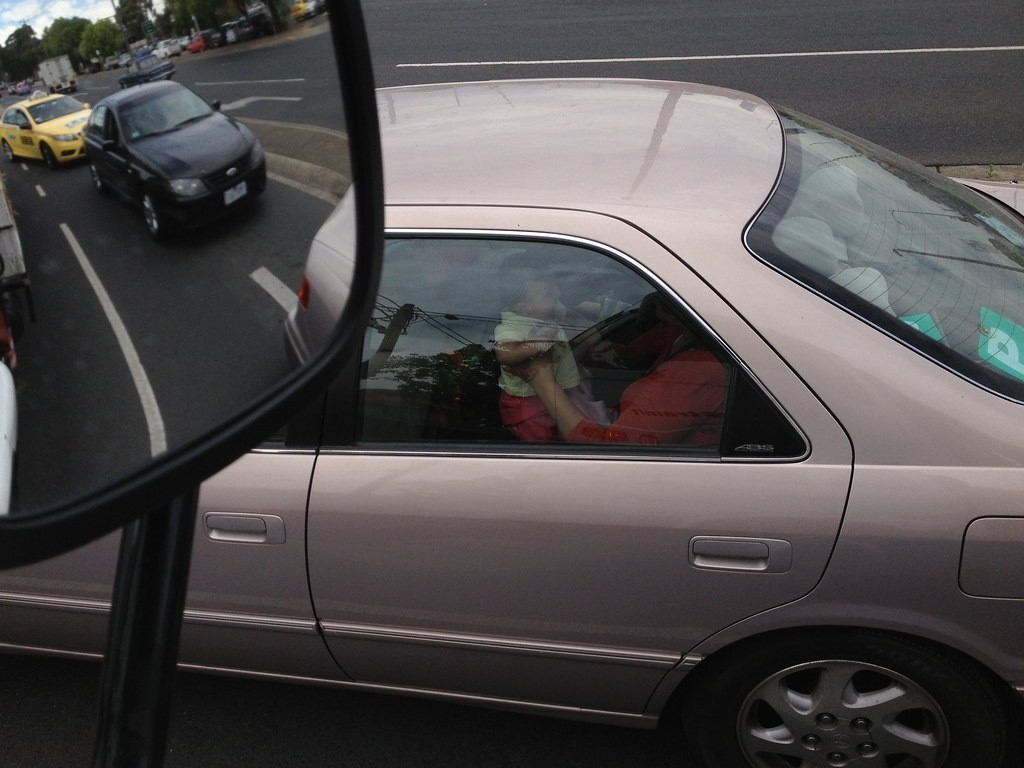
[36,55,80,95]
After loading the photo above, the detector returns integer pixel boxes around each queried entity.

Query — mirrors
[0,0,358,524]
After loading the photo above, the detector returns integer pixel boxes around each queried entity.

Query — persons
[492,251,582,441]
[574,298,633,323]
[499,301,726,446]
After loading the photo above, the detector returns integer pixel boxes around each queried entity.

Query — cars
[16,82,32,94]
[284,183,362,373]
[2,76,1022,768]
[87,0,323,71]
[83,82,267,237]
[2,91,96,168]
[115,54,178,91]
